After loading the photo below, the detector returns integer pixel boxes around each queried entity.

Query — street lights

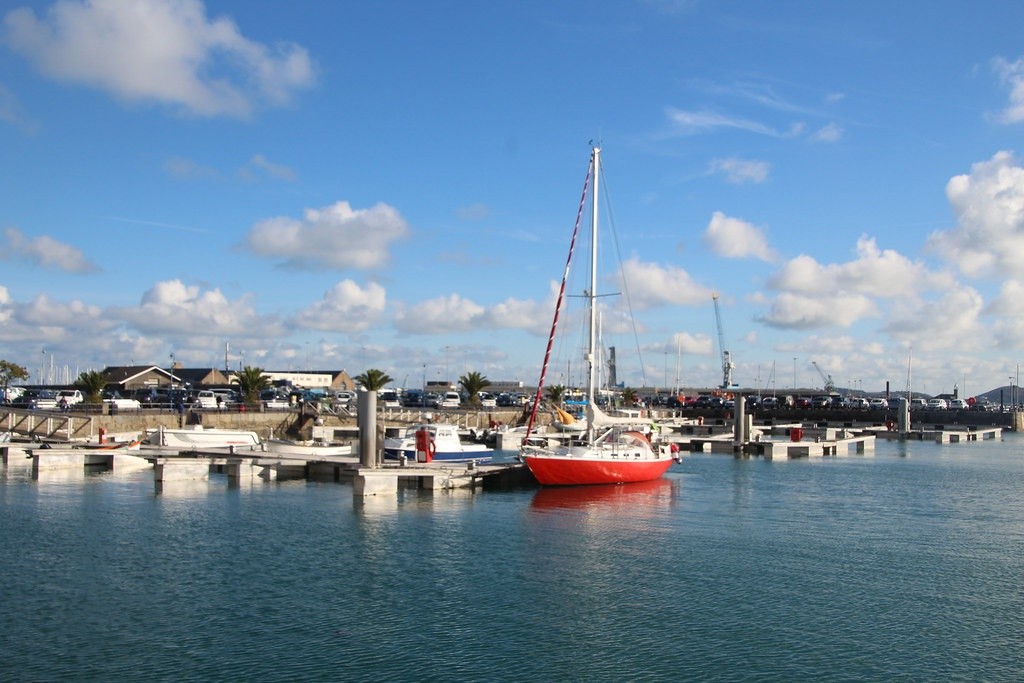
[793,357,796,390]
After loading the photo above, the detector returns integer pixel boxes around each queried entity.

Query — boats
[262,425,352,456]
[68,422,265,451]
[385,419,548,465]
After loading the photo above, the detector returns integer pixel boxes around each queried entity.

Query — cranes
[812,362,836,394]
[712,291,734,388]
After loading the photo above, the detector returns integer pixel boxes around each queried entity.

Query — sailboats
[517,141,683,484]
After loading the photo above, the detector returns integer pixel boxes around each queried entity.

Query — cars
[653,394,971,411]
[0,382,537,411]
